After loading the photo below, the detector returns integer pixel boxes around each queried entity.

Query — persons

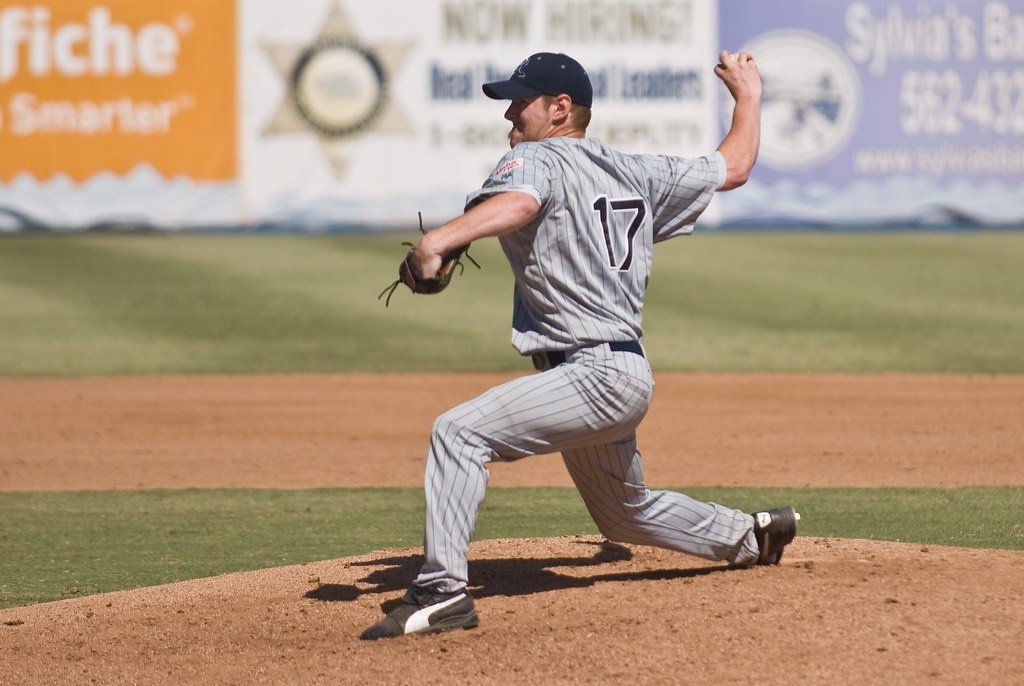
[358,50,800,640]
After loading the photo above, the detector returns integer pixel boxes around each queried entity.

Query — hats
[482,52,593,109]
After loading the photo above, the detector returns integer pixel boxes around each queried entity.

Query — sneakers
[751,505,796,565]
[361,586,479,640]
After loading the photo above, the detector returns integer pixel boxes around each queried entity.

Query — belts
[531,341,645,371]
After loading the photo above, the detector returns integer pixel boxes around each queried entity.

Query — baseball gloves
[399,240,471,294]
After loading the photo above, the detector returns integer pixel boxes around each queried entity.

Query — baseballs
[729,54,740,63]
